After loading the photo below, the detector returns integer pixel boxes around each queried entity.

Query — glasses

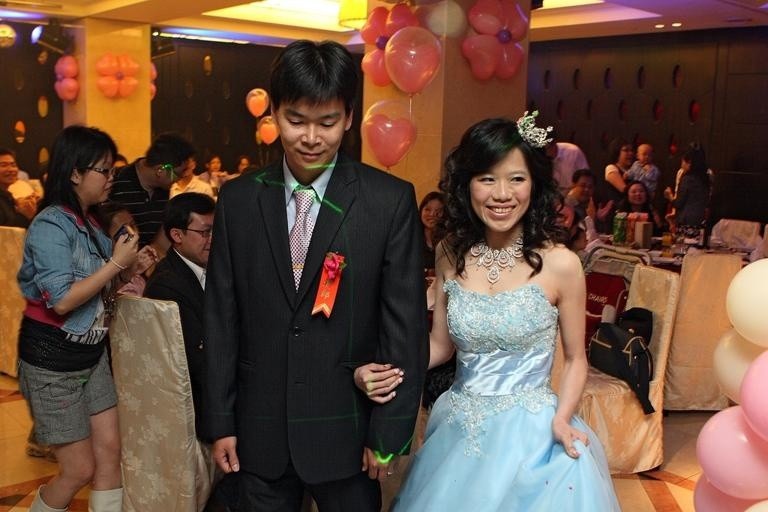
[89,166,116,176]
[621,148,635,152]
[182,228,212,238]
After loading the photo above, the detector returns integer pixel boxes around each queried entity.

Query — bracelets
[106,255,131,273]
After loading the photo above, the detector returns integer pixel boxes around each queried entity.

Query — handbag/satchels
[588,321,655,416]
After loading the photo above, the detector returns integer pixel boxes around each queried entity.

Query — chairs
[575,262,681,475]
[0,225,29,379]
[664,253,743,415]
[711,218,761,249]
[582,243,652,361]
[107,291,222,512]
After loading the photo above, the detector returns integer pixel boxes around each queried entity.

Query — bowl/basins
[599,235,611,241]
[648,251,662,257]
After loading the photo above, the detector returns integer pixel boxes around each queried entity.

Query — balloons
[258,117,280,145]
[725,258,768,349]
[386,28,442,96]
[694,471,768,512]
[739,349,768,443]
[246,88,270,119]
[96,52,141,102]
[712,328,766,402]
[461,1,529,82]
[360,99,418,173]
[54,55,81,103]
[360,3,419,89]
[151,60,159,104]
[695,405,768,499]
[746,499,768,512]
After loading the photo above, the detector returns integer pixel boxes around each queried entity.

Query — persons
[621,180,653,213]
[198,154,228,195]
[201,39,429,512]
[663,149,710,245]
[542,129,588,196]
[169,152,214,200]
[418,191,447,268]
[600,137,635,234]
[88,203,145,297]
[563,169,597,250]
[142,193,216,441]
[622,144,664,228]
[14,125,140,512]
[87,133,194,253]
[223,154,250,182]
[352,118,621,512]
[0,147,42,230]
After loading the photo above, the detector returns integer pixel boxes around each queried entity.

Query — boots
[29,484,68,512]
[87,486,124,512]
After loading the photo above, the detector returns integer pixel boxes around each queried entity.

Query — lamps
[30,24,68,56]
[150,43,178,61]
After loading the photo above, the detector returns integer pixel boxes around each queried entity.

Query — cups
[662,247,673,257]
[662,232,672,247]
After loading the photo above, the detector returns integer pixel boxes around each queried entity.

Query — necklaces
[455,225,534,287]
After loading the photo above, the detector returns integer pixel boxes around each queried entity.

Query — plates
[652,257,676,264]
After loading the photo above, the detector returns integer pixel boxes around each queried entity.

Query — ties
[289,185,316,293]
